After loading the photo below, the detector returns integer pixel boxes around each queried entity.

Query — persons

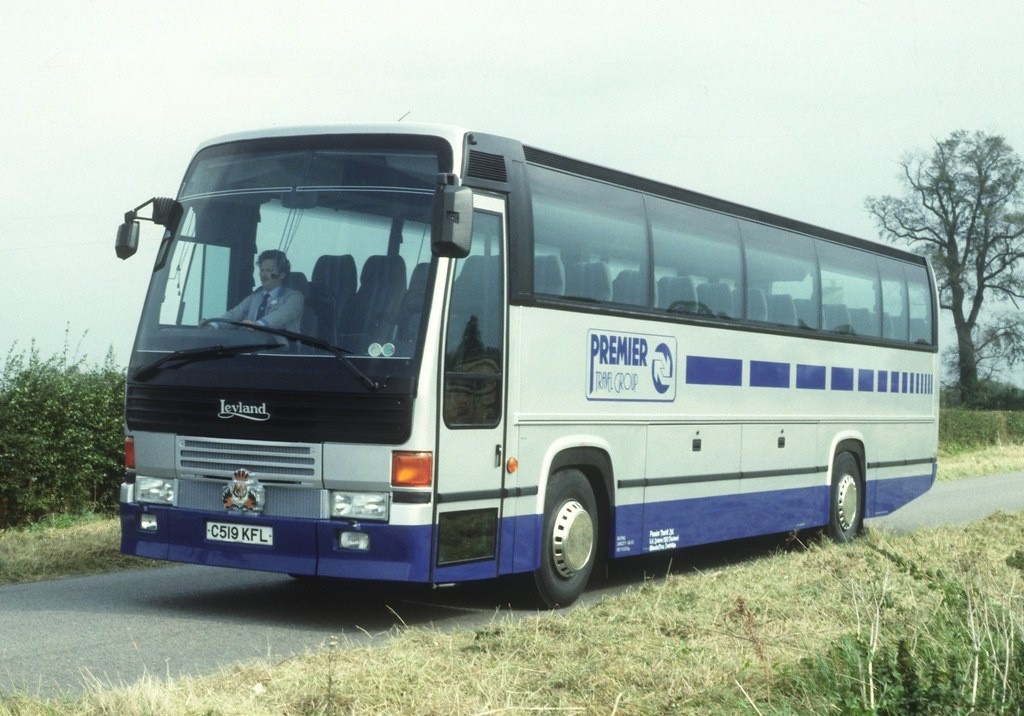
[198,250,305,354]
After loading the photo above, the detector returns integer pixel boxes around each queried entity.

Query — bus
[112,123,941,608]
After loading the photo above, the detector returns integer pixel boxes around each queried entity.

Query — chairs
[448,254,500,352]
[821,302,851,335]
[288,272,340,356]
[533,256,566,296]
[732,288,769,322]
[794,298,826,330]
[389,262,431,356]
[871,311,895,339]
[659,277,698,312]
[613,270,659,308]
[564,260,614,301]
[765,294,798,326]
[312,252,356,323]
[909,319,929,343]
[356,254,407,347]
[693,281,734,317]
[892,316,912,343]
[848,307,874,337]
